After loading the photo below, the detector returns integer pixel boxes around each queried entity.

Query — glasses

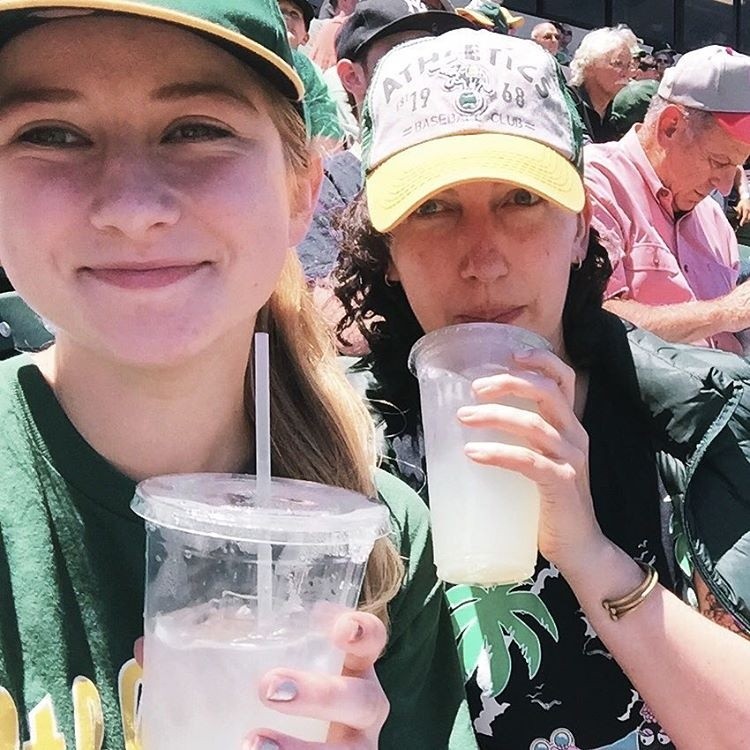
[657,59,670,64]
[652,65,658,70]
[543,33,560,40]
[562,30,572,35]
[421,1,439,10]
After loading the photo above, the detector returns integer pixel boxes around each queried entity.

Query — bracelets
[601,558,658,621]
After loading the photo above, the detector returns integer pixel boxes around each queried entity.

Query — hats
[440,0,525,28]
[657,45,750,143]
[612,79,660,133]
[334,0,478,62]
[652,40,677,57]
[359,28,586,232]
[631,46,643,58]
[0,1,305,102]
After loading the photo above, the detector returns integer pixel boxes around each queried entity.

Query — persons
[0,0,750,750]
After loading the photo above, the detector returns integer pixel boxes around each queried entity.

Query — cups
[408,321,553,587]
[130,471,392,750]
